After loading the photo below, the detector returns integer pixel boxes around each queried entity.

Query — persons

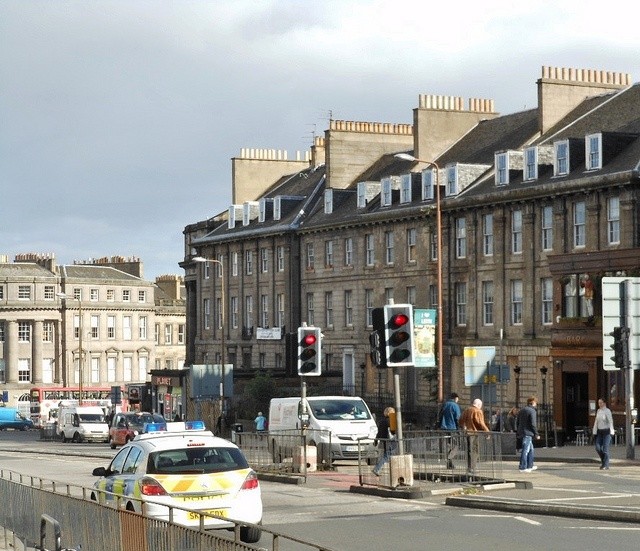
[591,397,616,469]
[118,416,124,426]
[488,408,505,432]
[438,392,462,469]
[370,407,395,474]
[253,412,266,431]
[516,396,541,473]
[458,398,491,472]
[173,414,180,421]
[506,406,522,453]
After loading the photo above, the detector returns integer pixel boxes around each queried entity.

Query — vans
[267,395,379,464]
[0,406,33,430]
[56,405,109,443]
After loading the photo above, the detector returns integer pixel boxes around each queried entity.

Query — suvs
[108,410,166,449]
[89,419,263,542]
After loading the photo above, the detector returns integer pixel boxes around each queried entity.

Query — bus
[30,385,131,428]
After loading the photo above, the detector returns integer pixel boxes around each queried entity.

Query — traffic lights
[298,326,322,376]
[384,303,415,368]
[369,330,382,364]
[609,326,624,368]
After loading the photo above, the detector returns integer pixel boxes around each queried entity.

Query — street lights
[539,364,547,408]
[191,255,226,437]
[57,292,83,406]
[512,365,521,408]
[393,152,443,427]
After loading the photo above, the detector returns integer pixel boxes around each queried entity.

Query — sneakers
[528,466,537,470]
[520,468,531,472]
